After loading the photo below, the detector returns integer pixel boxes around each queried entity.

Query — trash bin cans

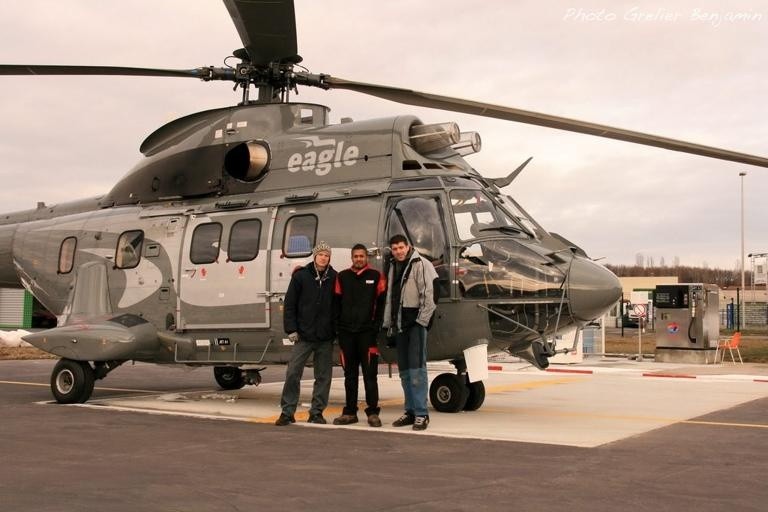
[582,325,601,358]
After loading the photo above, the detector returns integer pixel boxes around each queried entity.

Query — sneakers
[368,414,381,426]
[276,413,295,426]
[308,415,326,424]
[413,415,429,430]
[334,415,358,424]
[392,412,415,426]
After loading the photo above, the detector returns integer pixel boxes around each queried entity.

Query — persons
[381,235,440,431]
[275,241,337,426]
[330,243,388,427]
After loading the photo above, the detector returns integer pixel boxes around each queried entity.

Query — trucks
[624,302,648,328]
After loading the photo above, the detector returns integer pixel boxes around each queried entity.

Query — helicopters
[0,1,768,414]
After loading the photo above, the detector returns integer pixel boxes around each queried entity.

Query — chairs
[713,332,745,367]
[287,235,312,253]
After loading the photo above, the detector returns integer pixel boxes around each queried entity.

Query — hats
[312,241,331,281]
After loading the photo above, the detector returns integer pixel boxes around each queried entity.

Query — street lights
[736,171,749,330]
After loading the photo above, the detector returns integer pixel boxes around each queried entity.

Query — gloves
[288,332,298,342]
